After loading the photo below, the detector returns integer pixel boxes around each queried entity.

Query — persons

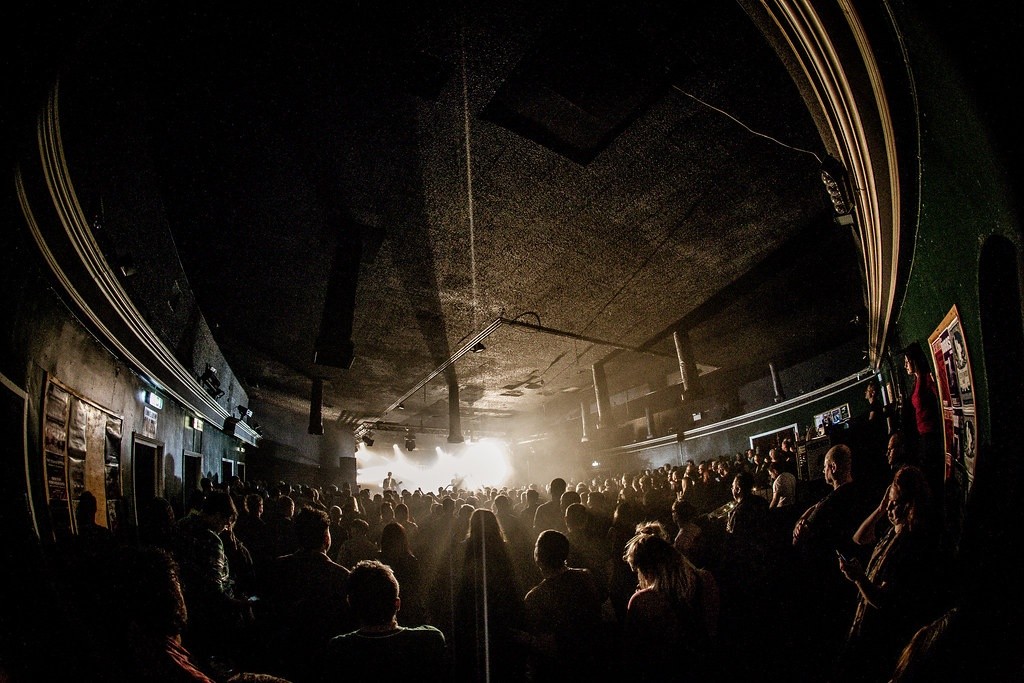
[903,342,943,462]
[1,414,1023,683]
[863,383,882,421]
